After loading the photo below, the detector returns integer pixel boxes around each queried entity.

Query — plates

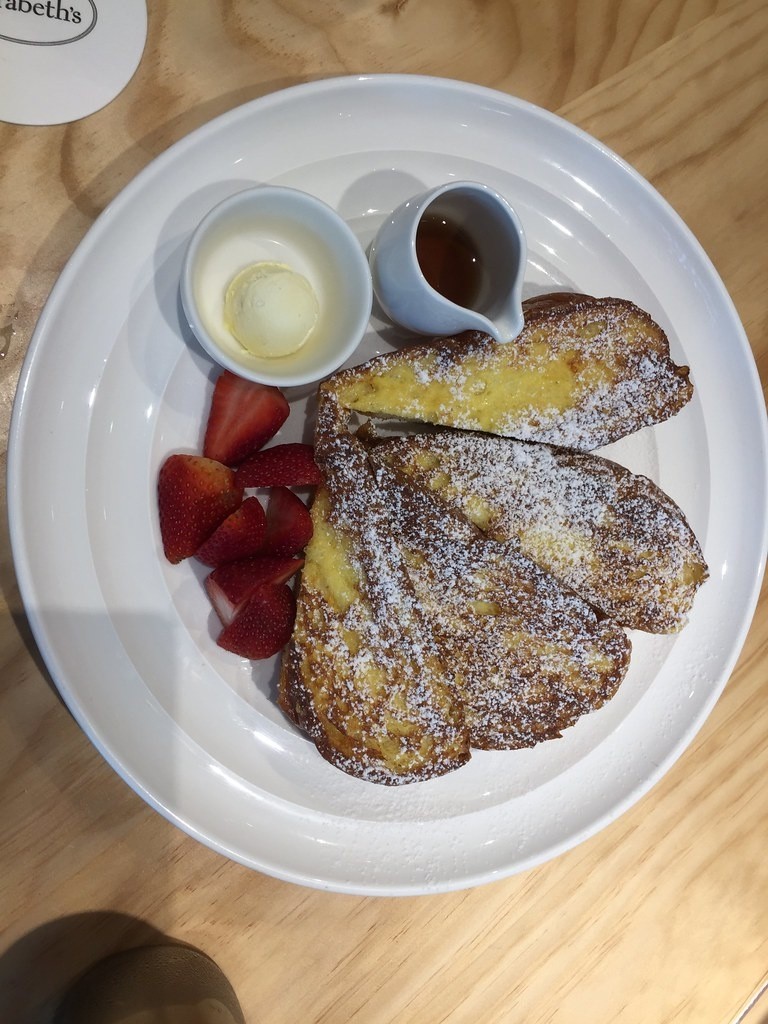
[5,72,768,897]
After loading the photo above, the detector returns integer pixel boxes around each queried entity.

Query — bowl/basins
[178,184,373,388]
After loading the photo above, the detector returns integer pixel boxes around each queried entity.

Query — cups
[367,178,527,346]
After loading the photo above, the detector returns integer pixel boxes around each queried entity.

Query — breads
[272,293,708,787]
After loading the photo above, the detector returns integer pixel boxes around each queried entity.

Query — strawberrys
[158,371,323,660]
